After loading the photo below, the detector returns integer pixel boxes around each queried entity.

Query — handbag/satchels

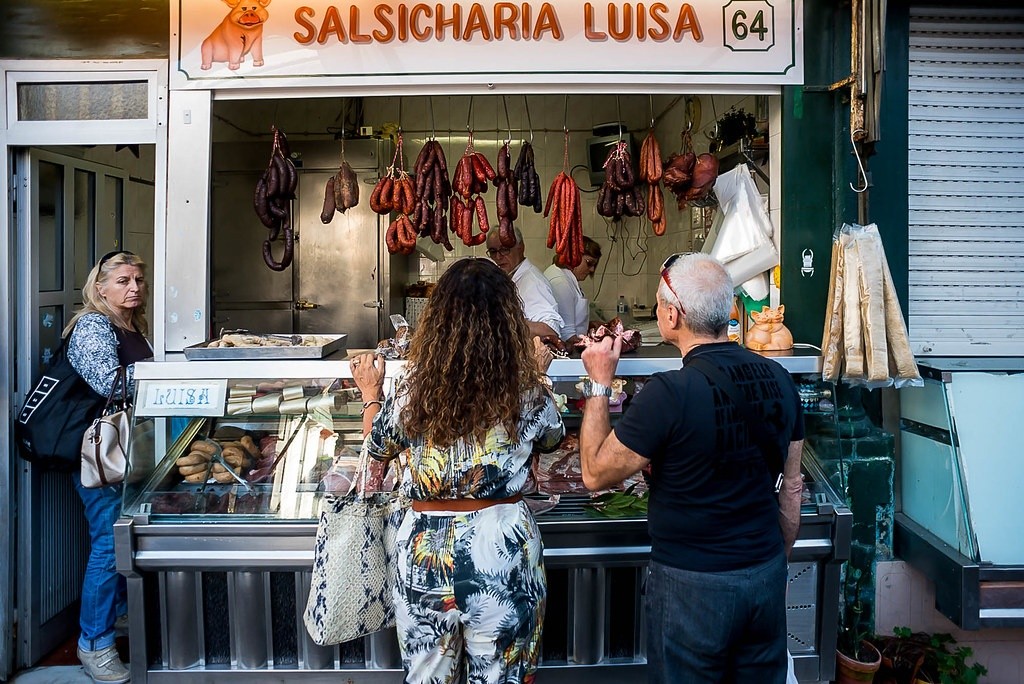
[301,438,408,646]
[81,367,154,488]
[18,316,124,468]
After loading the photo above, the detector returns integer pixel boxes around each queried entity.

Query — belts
[412,493,524,510]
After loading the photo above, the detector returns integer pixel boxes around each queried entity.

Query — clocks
[684,96,702,134]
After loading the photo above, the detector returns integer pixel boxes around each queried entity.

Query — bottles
[727,293,741,346]
[616,295,628,327]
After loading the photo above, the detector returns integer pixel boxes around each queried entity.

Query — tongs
[219,328,303,346]
[195,453,256,513]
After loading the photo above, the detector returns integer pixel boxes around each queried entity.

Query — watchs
[582,377,612,399]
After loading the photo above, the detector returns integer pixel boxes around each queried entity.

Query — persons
[580,252,805,684]
[350,256,566,684]
[485,225,565,339]
[61,251,154,684]
[543,236,601,345]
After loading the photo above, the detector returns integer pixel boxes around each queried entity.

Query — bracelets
[360,401,384,416]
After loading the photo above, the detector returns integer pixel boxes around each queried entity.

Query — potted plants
[836,558,883,684]
[717,105,756,147]
[876,626,957,684]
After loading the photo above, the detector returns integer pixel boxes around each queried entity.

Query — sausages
[370,139,584,270]
[252,154,298,270]
[175,436,259,483]
[596,134,666,235]
[320,162,360,224]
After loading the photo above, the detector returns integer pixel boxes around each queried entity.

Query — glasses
[97,251,133,282]
[582,255,595,268]
[485,247,511,258]
[660,251,693,314]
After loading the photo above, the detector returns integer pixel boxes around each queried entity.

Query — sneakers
[77,644,129,684]
[114,613,130,637]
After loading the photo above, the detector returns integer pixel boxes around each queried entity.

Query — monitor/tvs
[586,132,639,186]
